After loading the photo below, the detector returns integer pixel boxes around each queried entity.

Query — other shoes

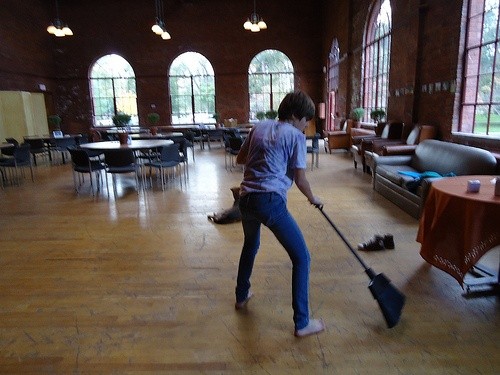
[356,233,385,251]
[383,233,395,250]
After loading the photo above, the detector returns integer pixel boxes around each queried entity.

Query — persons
[235,90,325,338]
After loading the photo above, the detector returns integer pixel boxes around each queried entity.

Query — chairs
[0,123,249,202]
[306,132,320,169]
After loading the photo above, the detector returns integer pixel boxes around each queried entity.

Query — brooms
[316,205,406,329]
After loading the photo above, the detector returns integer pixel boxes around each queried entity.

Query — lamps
[151,0,171,40]
[47,0,74,36]
[244,0,267,32]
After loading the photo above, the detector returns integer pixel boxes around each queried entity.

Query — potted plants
[112,112,132,144]
[147,114,159,136]
[353,107,365,129]
[370,109,386,133]
[212,113,221,127]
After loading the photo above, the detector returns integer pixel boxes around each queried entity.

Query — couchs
[323,119,497,220]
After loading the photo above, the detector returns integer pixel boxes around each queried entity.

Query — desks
[80,139,173,150]
[416,175,500,294]
[130,132,183,139]
[23,134,82,166]
